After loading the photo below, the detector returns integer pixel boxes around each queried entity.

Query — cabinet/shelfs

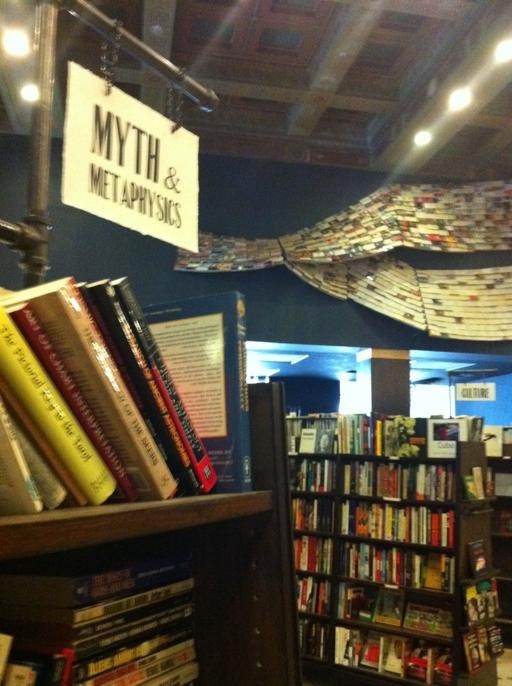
[486,443,512,650]
[1,378,302,686]
[291,442,497,686]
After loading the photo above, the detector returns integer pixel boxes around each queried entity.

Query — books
[339,582,455,638]
[292,493,336,534]
[337,497,459,548]
[333,625,456,686]
[336,459,458,499]
[463,627,505,670]
[294,537,334,574]
[342,540,455,593]
[296,619,332,664]
[288,456,337,492]
[465,576,509,625]
[467,539,488,576]
[287,416,457,459]
[295,576,336,617]
[464,466,486,499]
[1,554,276,686]
